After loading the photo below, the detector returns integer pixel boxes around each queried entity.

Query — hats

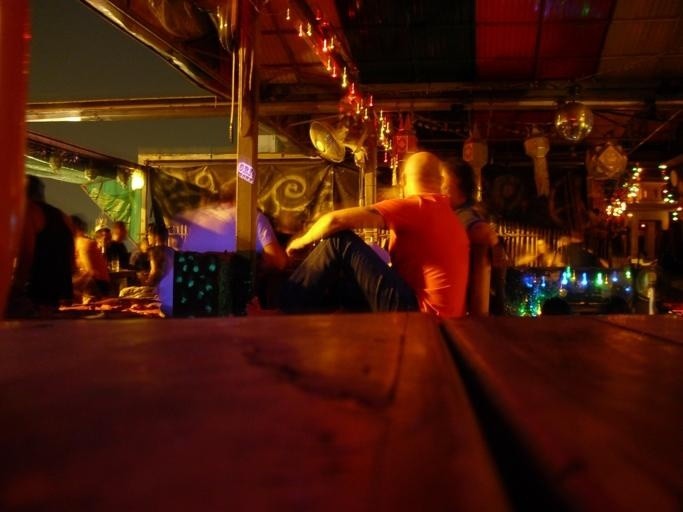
[95,224,111,232]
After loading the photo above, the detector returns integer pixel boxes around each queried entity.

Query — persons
[489,236,632,314]
[159,175,287,319]
[278,152,471,319]
[439,158,507,288]
[6,174,177,319]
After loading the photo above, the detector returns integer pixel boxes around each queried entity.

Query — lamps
[309,116,354,162]
[50,154,128,188]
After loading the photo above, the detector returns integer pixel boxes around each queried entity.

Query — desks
[441,315,683,512]
[507,265,633,315]
[0,312,508,512]
[110,270,138,294]
